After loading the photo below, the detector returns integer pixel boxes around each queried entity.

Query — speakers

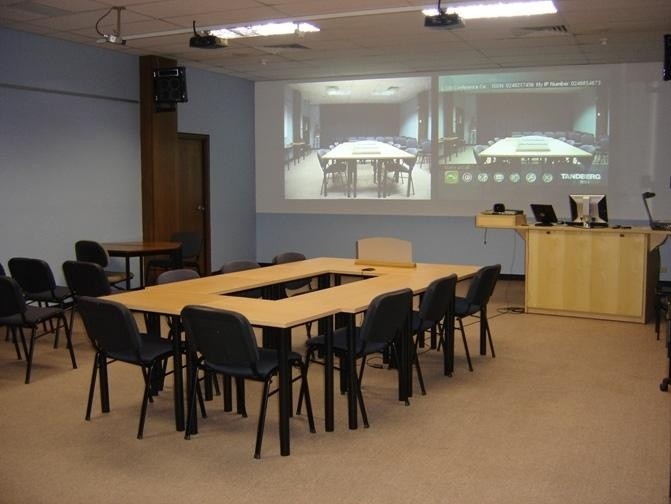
[664,33,671,79]
[152,67,188,104]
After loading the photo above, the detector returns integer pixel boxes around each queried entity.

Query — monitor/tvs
[529,204,558,226]
[569,194,608,227]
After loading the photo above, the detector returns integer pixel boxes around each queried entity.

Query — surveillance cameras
[102,35,127,46]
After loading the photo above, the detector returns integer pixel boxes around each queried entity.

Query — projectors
[424,14,466,29]
[190,36,229,48]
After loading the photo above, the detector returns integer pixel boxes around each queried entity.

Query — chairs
[75,238,135,293]
[157,266,223,396]
[294,287,413,431]
[414,272,458,396]
[61,258,112,299]
[222,259,267,299]
[180,303,318,460]
[75,295,186,440]
[436,263,500,373]
[8,254,72,350]
[473,129,597,175]
[317,135,431,199]
[271,250,320,361]
[0,263,77,385]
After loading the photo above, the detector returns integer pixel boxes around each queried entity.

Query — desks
[103,241,182,292]
[277,273,458,430]
[283,142,311,171]
[94,287,343,458]
[475,208,671,322]
[301,255,486,378]
[439,136,460,164]
[143,258,330,353]
